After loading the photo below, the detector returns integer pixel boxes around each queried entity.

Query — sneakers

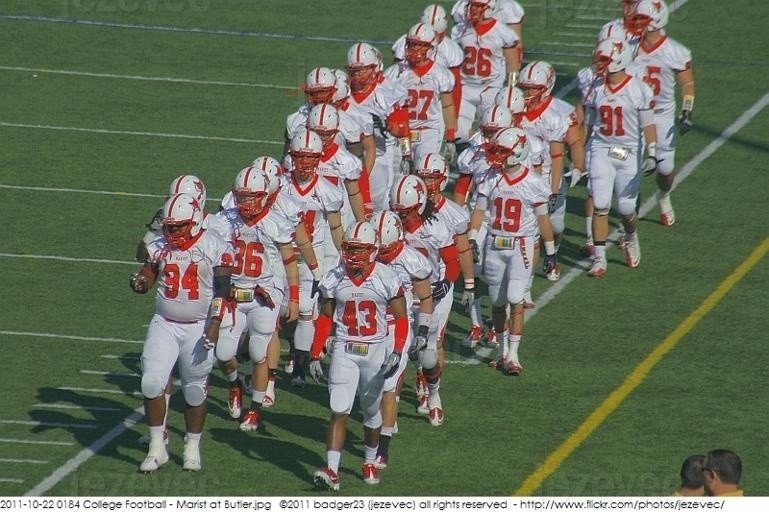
[230,376,275,432]
[313,468,340,491]
[182,439,202,471]
[285,359,306,386]
[461,323,523,375]
[655,191,676,226]
[416,369,444,426]
[362,453,387,485]
[581,233,641,277]
[524,288,535,308]
[546,264,560,281]
[138,431,170,473]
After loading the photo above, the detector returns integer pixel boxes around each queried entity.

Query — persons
[701,449,746,496]
[670,452,708,496]
[128,0,697,489]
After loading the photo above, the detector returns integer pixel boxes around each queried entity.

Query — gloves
[381,351,401,379]
[543,254,556,273]
[410,333,428,353]
[461,288,474,313]
[468,239,480,264]
[678,110,692,134]
[563,169,582,188]
[309,359,323,385]
[431,279,450,300]
[311,280,322,301]
[641,156,657,177]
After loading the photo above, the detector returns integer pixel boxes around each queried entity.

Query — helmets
[591,0,668,78]
[159,0,556,269]
